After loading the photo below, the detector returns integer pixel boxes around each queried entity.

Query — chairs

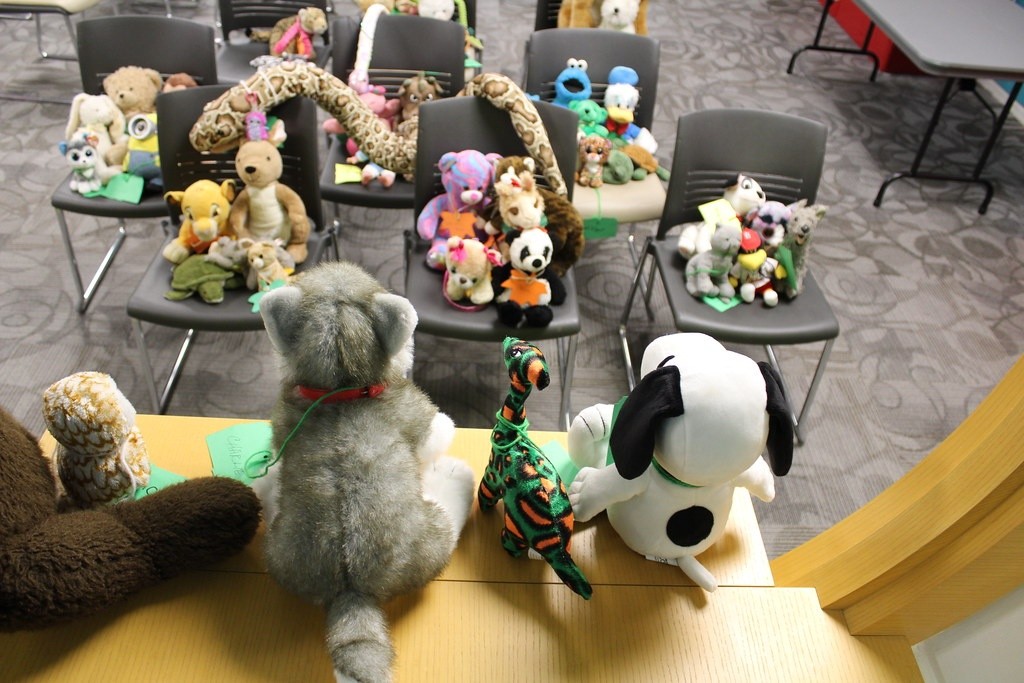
[0,0,840,441]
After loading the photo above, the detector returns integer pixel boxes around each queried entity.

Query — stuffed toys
[566,334,794,592]
[164,114,310,303]
[60,67,195,192]
[418,149,584,325]
[559,0,647,36]
[322,4,440,186]
[42,371,150,507]
[476,340,592,601]
[190,62,568,197]
[553,58,670,187]
[0,405,262,631]
[257,265,474,683]
[245,7,326,59]
[676,173,827,306]
[358,0,483,67]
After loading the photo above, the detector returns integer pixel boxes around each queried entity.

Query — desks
[785,0,1024,215]
[0,351,1024,682]
